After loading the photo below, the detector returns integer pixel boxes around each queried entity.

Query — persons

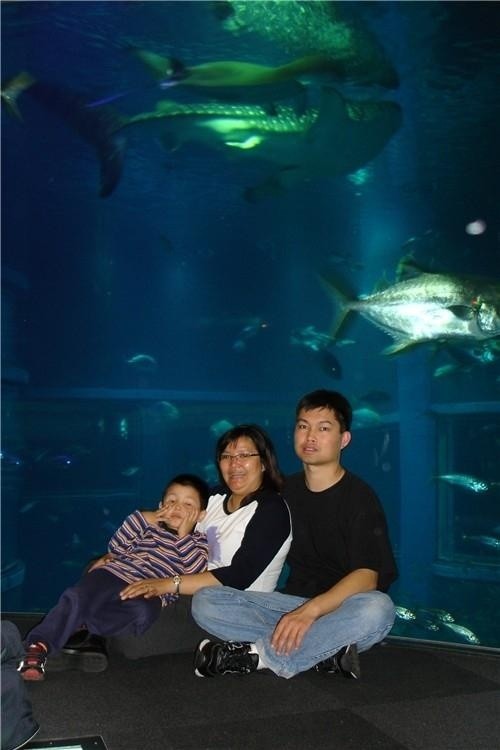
[62,425,294,673]
[191,390,399,682]
[16,474,210,682]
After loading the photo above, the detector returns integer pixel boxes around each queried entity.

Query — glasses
[221,452,260,461]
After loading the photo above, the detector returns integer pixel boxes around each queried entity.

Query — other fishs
[1,44,401,208]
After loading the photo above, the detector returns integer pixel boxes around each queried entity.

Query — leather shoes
[45,630,108,675]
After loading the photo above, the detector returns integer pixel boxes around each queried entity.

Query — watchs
[173,574,182,593]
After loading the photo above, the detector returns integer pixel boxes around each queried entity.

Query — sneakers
[16,640,48,681]
[316,642,363,680]
[193,635,260,679]
